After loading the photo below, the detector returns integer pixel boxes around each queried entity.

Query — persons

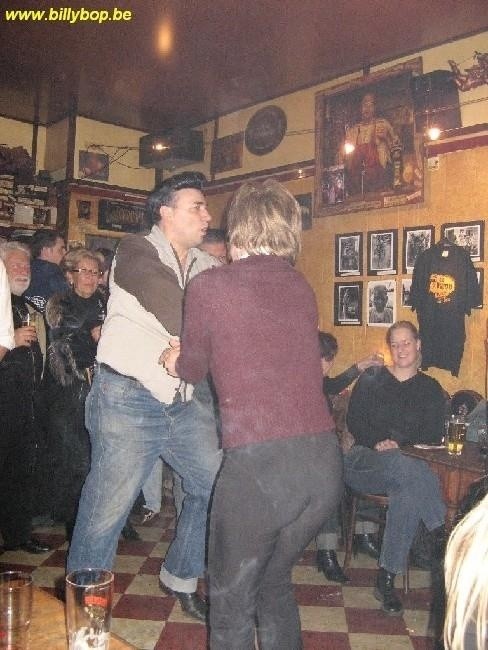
[443,470,487,647]
[0,239,54,554]
[341,93,403,192]
[89,248,116,288]
[312,330,381,586]
[337,319,452,610]
[197,227,231,267]
[66,175,227,623]
[159,178,345,649]
[22,228,70,344]
[47,247,141,542]
[1,259,16,359]
[369,286,393,325]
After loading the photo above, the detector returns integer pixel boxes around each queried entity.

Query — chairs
[341,376,409,593]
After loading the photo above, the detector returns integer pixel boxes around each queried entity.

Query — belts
[99,362,140,383]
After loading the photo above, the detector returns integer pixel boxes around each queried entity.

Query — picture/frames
[333,280,363,327]
[402,224,436,275]
[473,268,484,310]
[440,219,485,265]
[335,232,363,275]
[366,229,398,275]
[313,53,429,218]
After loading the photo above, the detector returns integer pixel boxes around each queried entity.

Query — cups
[447,414,467,457]
[375,353,385,367]
[478,430,488,467]
[22,313,36,328]
[1,569,34,649]
[63,567,116,650]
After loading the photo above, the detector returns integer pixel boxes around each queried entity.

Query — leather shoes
[371,567,406,617]
[18,537,52,554]
[352,533,381,560]
[156,579,208,624]
[316,548,352,587]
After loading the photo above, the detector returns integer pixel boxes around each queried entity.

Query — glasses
[69,267,103,278]
